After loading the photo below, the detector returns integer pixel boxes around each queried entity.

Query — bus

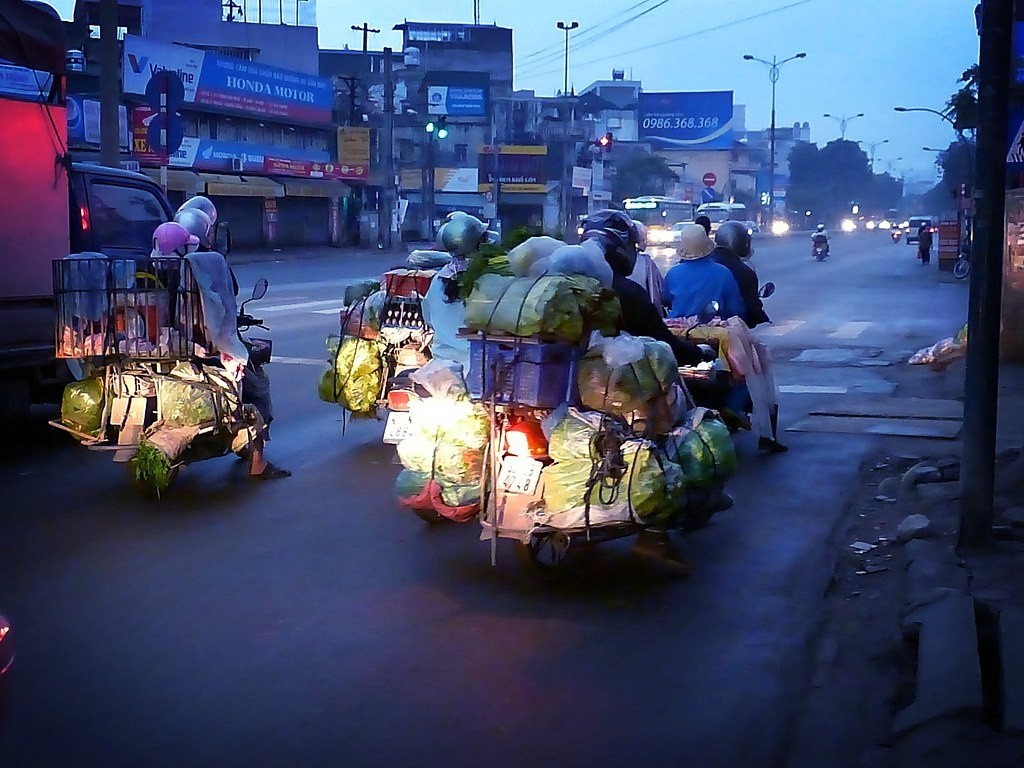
[696,202,746,244]
[621,196,692,248]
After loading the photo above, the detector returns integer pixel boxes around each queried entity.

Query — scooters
[689,281,777,437]
[135,278,273,496]
[383,273,723,564]
[812,234,830,263]
[891,230,902,244]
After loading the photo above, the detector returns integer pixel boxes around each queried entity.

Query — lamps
[229,157,244,174]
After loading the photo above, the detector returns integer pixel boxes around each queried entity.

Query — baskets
[383,296,423,329]
[242,338,272,367]
[385,272,435,296]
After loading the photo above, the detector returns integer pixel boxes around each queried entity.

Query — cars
[747,221,759,238]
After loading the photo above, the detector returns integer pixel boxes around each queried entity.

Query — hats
[676,224,716,260]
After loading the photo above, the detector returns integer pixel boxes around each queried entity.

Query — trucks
[0,0,233,442]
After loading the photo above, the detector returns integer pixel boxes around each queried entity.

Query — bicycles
[953,258,971,279]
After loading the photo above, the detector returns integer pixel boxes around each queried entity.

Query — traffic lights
[425,122,436,134]
[438,113,447,138]
[606,133,613,152]
[761,191,767,205]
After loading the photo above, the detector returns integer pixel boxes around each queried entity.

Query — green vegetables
[452,224,619,342]
[397,341,738,524]
[61,362,223,491]
[318,335,385,414]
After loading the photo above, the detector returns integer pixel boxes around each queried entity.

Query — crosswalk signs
[1003,113,1024,172]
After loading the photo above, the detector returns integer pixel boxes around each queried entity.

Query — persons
[959,214,969,246]
[149,195,292,478]
[577,209,716,576]
[661,216,790,452]
[420,211,497,368]
[918,222,932,265]
[810,223,829,252]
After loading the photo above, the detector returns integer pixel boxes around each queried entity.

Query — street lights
[556,20,580,235]
[893,107,971,244]
[857,139,889,173]
[823,113,864,141]
[744,53,807,232]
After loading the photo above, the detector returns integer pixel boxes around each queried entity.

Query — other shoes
[725,406,751,429]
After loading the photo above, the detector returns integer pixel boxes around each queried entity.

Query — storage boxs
[466,331,583,408]
[110,291,171,347]
[386,269,437,297]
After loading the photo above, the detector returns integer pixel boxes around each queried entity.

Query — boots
[759,404,789,451]
[631,529,693,574]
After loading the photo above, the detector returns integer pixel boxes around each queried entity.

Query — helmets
[173,209,212,248]
[152,222,200,255]
[581,209,639,276]
[715,221,751,256]
[632,220,648,252]
[444,211,468,222]
[442,215,489,254]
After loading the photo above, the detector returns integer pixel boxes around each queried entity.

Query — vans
[906,216,934,244]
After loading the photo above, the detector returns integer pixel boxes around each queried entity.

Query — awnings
[268,176,350,198]
[196,173,285,197]
[140,169,206,193]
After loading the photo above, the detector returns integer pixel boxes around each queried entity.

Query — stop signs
[701,172,716,186]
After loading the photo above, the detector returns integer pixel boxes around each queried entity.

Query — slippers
[247,461,291,480]
